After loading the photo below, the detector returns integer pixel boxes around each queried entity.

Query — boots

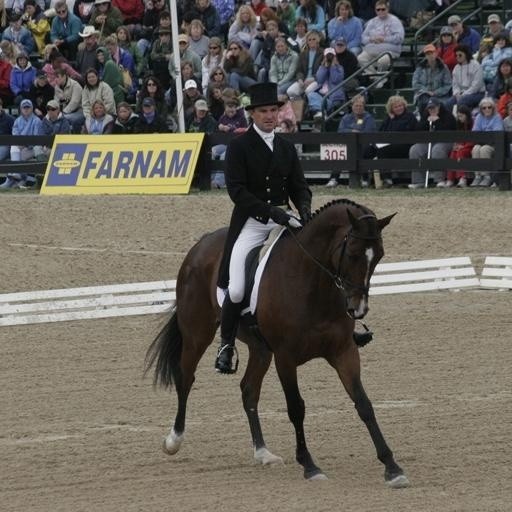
[215,291,244,370]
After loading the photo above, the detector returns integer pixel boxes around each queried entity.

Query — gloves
[270,207,290,225]
[299,204,311,221]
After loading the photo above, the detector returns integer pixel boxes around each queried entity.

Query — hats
[20,99,32,108]
[178,34,189,44]
[423,15,460,53]
[47,100,58,109]
[324,37,344,56]
[185,80,197,90]
[426,98,440,110]
[488,14,500,24]
[156,27,171,34]
[195,100,208,111]
[78,26,99,37]
[143,97,153,107]
[245,83,285,110]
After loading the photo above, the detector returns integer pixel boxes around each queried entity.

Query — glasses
[482,106,491,109]
[209,47,237,76]
[376,8,388,11]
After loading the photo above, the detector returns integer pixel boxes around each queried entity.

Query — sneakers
[408,177,491,189]
[326,179,338,187]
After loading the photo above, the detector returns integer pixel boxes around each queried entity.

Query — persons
[214,83,373,371]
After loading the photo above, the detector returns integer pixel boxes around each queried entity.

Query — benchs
[1,1,511,132]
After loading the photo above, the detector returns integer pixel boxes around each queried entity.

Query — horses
[142,197,413,488]
